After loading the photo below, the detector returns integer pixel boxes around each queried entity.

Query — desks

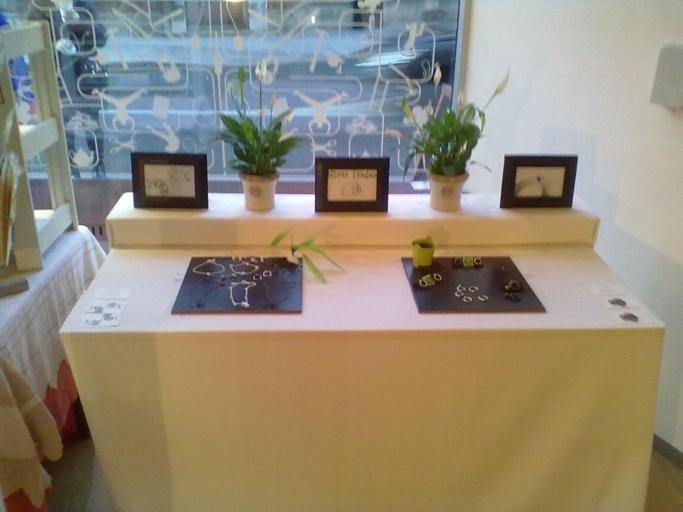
[0,223,109,512]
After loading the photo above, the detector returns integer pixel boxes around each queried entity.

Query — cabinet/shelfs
[59,191,666,512]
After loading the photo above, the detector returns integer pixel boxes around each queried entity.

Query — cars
[400,29,456,85]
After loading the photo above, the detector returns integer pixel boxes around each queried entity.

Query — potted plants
[391,65,510,212]
[213,62,302,212]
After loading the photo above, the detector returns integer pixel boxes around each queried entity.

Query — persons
[25,1,107,179]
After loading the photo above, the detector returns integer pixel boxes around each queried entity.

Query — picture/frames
[130,150,209,211]
[314,156,390,213]
[501,153,578,208]
